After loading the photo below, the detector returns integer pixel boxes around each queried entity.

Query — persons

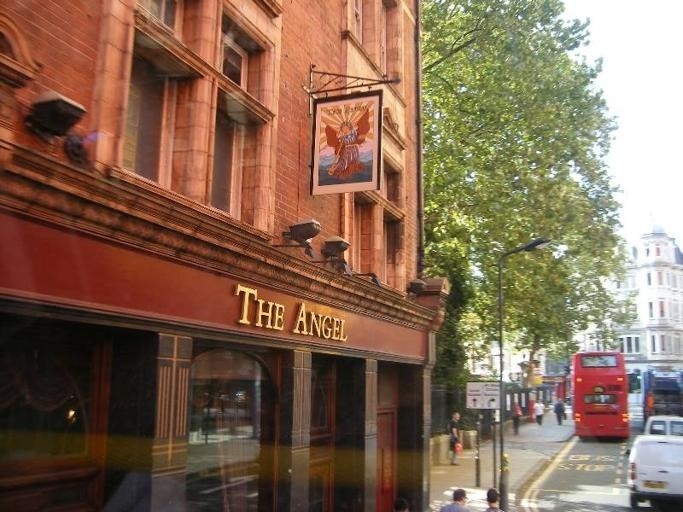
[440,489,469,512]
[447,410,461,466]
[510,401,523,436]
[394,498,408,512]
[554,397,565,425]
[325,109,370,179]
[483,488,504,512]
[532,399,545,426]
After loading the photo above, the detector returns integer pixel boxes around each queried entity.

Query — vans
[626,436,683,509]
[644,415,682,437]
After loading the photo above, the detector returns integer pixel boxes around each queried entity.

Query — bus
[569,350,630,440]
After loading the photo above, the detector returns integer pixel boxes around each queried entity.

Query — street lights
[496,239,552,512]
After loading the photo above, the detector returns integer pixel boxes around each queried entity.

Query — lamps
[407,279,426,293]
[321,236,351,256]
[284,220,322,243]
[26,92,86,145]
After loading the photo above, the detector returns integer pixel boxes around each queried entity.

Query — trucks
[642,366,682,424]
